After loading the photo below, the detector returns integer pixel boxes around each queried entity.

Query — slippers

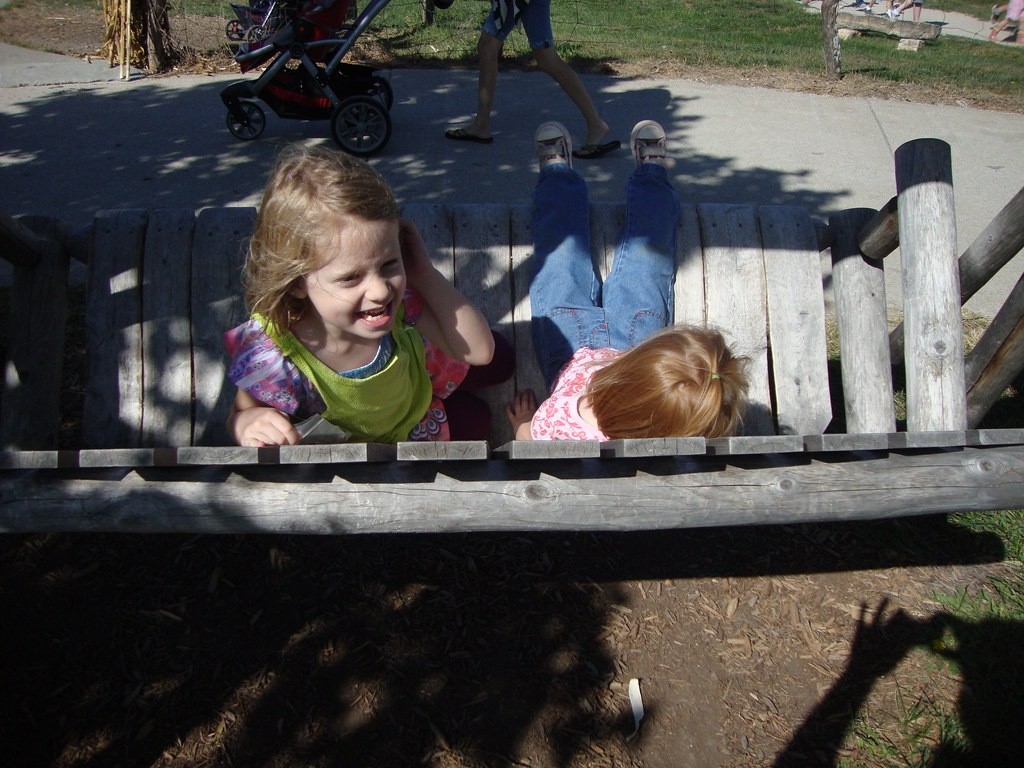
[445,128,493,143]
[575,140,621,159]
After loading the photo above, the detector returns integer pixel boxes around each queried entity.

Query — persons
[445,1,620,158]
[989,0,1024,44]
[505,119,752,444]
[864,0,923,22]
[227,147,495,446]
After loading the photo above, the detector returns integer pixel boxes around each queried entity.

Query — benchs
[0,137,1024,533]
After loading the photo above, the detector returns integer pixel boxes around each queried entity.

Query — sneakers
[630,119,668,169]
[534,121,572,171]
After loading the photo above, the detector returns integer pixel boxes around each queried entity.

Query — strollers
[220,0,452,155]
